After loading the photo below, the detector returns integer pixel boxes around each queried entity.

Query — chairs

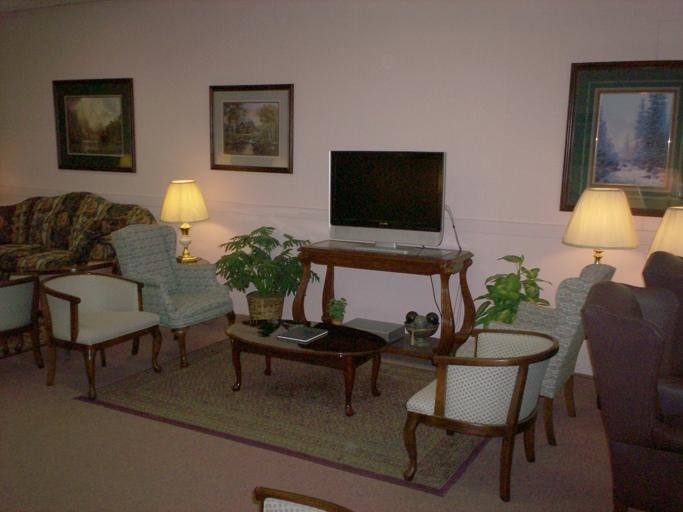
[0,275,45,369]
[401,325,561,502]
[579,280,683,510]
[108,222,237,370]
[641,249,682,292]
[442,264,615,446]
[251,481,353,512]
[36,271,165,401]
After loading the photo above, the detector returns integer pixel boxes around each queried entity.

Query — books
[276,325,328,344]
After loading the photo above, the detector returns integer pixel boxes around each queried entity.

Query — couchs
[0,190,160,322]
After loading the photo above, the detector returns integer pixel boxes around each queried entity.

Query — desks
[291,239,476,370]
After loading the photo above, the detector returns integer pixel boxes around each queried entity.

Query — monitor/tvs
[329,149,447,253]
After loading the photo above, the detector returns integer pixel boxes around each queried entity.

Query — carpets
[0,318,48,362]
[71,331,491,500]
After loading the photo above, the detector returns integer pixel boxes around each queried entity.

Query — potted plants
[327,297,347,325]
[214,225,323,328]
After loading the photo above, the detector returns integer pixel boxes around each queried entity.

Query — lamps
[558,186,640,265]
[641,206,682,267]
[159,178,211,263]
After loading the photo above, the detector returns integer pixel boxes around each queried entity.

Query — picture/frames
[50,76,138,174]
[207,82,296,175]
[557,59,683,218]
